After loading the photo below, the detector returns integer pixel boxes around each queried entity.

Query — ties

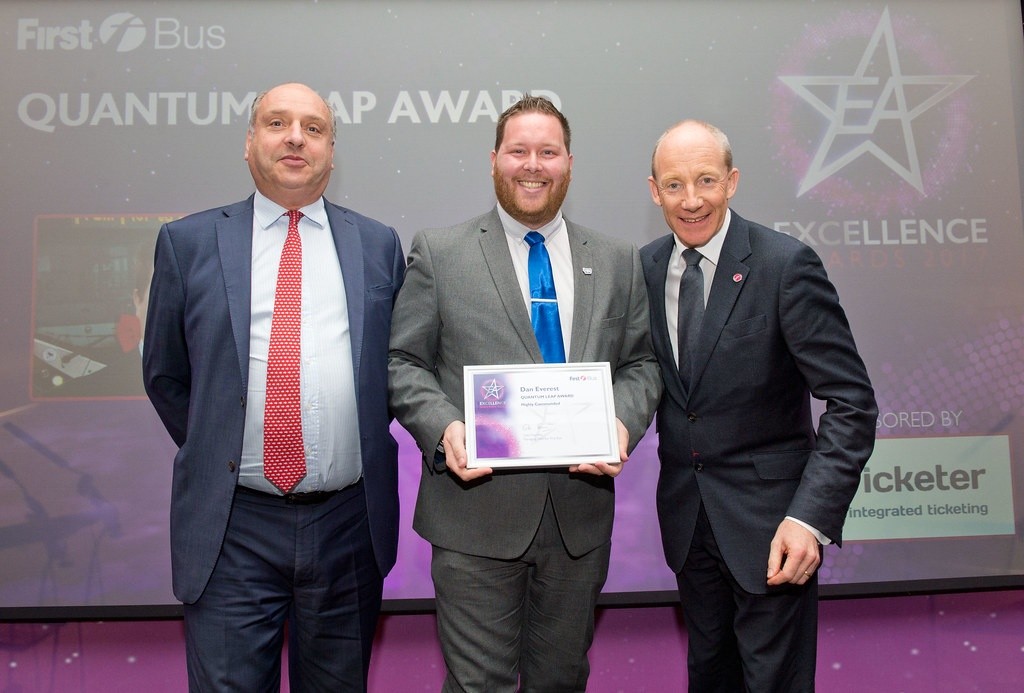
[524,231,567,364]
[263,210,307,495]
[678,248,705,394]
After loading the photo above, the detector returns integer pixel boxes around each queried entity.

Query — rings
[804,571,811,578]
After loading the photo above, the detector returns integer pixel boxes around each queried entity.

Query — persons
[639,119,879,693]
[388,92,664,693]
[142,84,406,693]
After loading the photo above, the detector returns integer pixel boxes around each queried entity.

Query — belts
[235,478,364,505]
